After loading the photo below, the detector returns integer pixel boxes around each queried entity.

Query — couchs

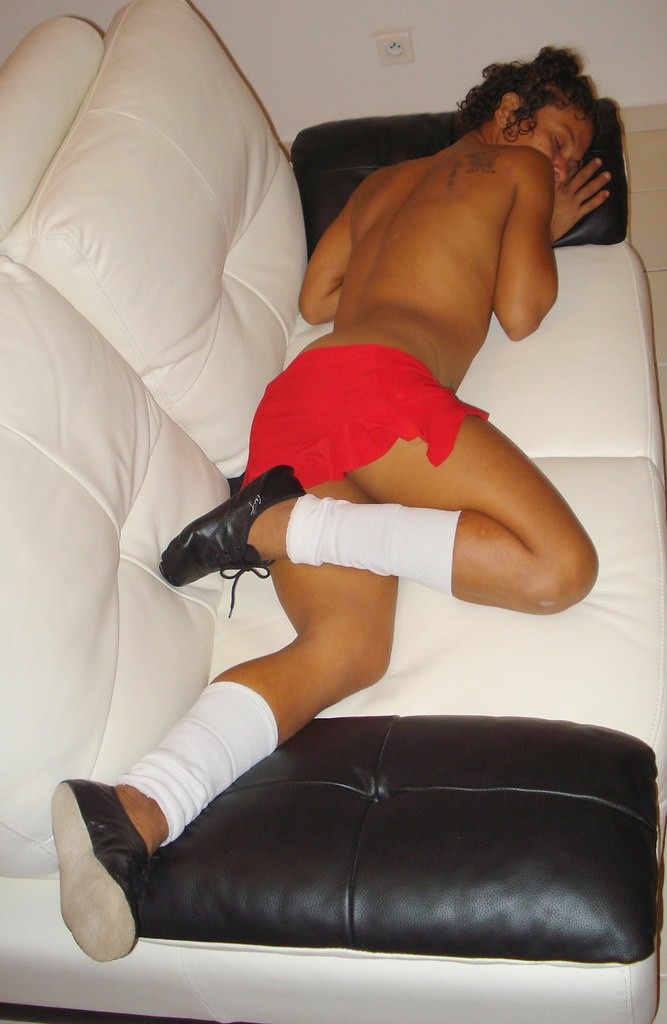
[0,0,667,1024]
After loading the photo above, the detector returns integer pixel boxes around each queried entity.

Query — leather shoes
[52,780,150,962]
[159,465,305,587]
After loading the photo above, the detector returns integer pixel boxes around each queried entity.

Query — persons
[50,46,613,964]
